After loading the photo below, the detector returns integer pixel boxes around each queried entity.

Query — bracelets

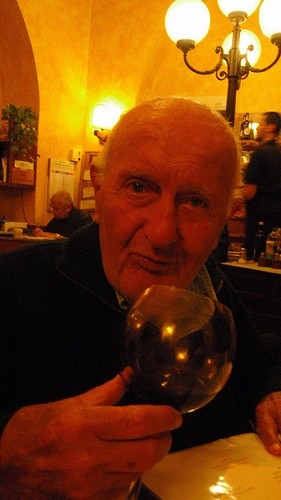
[55,233,60,238]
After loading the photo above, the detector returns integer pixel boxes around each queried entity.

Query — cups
[13,227,23,238]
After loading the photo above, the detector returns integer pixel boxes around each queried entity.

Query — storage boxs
[2,222,28,232]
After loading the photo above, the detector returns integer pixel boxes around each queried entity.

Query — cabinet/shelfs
[226,138,281,241]
[78,151,99,215]
[0,118,37,192]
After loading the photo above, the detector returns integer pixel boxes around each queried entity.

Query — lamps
[91,101,122,145]
[240,113,260,140]
[163,0,281,129]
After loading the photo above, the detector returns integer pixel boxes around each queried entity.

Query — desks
[137,432,281,500]
[220,259,281,361]
[0,227,69,255]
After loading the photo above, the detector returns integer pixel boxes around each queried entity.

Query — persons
[0,97,281,500]
[229,111,281,262]
[30,192,94,240]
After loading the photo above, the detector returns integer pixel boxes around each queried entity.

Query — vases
[12,160,35,185]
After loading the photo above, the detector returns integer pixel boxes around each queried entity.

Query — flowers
[1,104,38,162]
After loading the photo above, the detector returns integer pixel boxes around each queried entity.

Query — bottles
[243,113,251,140]
[238,247,247,264]
[239,114,246,140]
[254,221,281,262]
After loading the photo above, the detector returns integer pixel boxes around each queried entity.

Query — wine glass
[123,284,236,500]
